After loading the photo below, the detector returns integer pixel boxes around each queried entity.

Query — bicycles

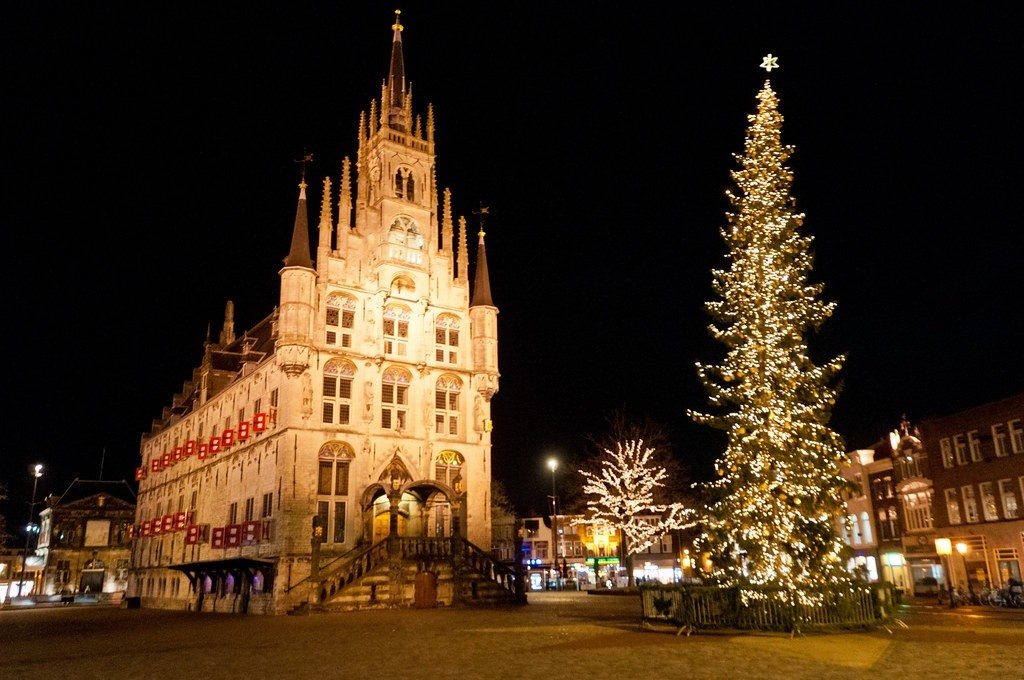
[951,578,1024,608]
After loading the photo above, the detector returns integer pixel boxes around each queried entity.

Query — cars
[913,577,939,597]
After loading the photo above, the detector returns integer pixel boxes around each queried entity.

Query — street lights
[16,463,44,597]
[935,537,957,609]
[548,459,560,591]
